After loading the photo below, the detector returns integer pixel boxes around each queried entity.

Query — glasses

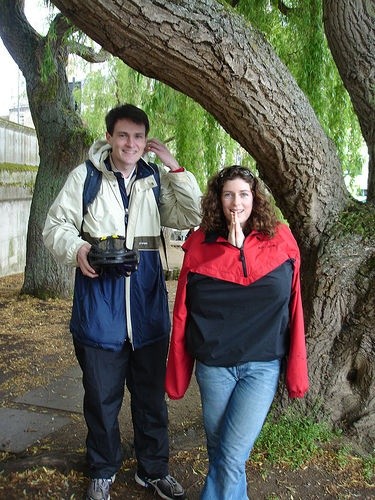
[219,166,255,179]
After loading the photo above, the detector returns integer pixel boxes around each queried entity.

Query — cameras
[162,267,180,281]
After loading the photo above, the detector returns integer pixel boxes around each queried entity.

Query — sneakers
[87,473,116,500]
[135,472,186,500]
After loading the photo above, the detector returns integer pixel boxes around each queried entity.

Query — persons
[164,165,309,497]
[41,103,205,500]
